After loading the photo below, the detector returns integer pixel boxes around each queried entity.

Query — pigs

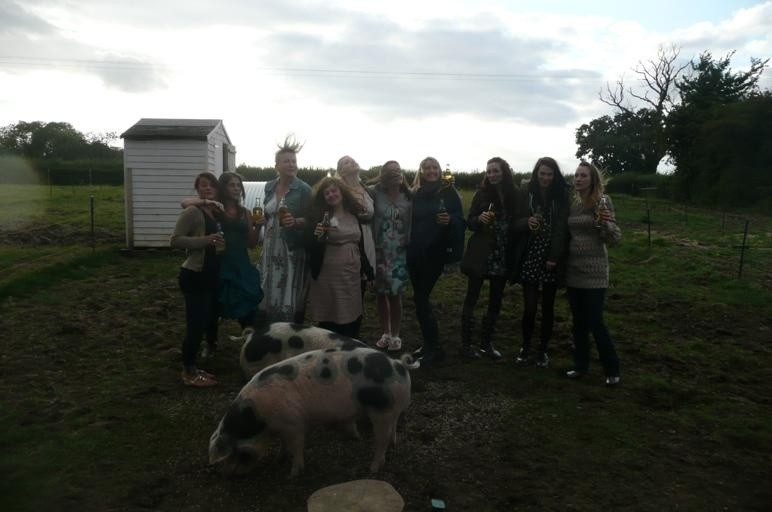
[228,322,372,442]
[207,348,426,486]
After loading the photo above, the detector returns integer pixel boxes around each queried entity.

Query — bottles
[215,223,226,253]
[252,198,262,226]
[318,208,329,241]
[278,195,288,226]
[597,198,607,217]
[533,205,543,228]
[439,198,447,224]
[488,202,496,216]
[443,169,451,185]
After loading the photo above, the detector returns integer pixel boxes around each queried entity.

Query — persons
[461,157,621,386]
[170,171,266,387]
[256,144,466,367]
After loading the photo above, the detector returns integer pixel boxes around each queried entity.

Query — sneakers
[376,335,389,348]
[387,337,402,351]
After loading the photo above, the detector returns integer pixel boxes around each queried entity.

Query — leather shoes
[513,346,530,367]
[535,349,551,367]
[184,375,218,386]
[567,370,583,378]
[463,343,481,360]
[605,375,619,386]
[198,370,215,379]
[481,343,501,359]
[416,344,442,363]
